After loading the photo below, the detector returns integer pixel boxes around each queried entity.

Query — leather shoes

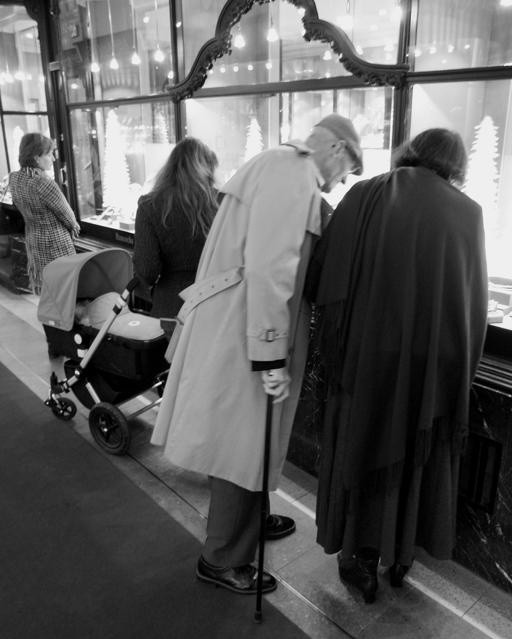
[196,553,276,594]
[265,514,296,540]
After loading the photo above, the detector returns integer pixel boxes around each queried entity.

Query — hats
[314,114,363,176]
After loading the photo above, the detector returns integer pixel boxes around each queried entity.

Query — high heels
[378,557,413,585]
[337,550,378,603]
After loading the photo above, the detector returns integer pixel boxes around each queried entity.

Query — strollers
[36,243,181,450]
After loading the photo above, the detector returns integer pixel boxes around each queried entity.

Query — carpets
[0,360,308,638]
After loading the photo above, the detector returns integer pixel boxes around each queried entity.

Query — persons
[8,132,80,359]
[303,127,490,604]
[131,137,227,343]
[149,113,366,596]
